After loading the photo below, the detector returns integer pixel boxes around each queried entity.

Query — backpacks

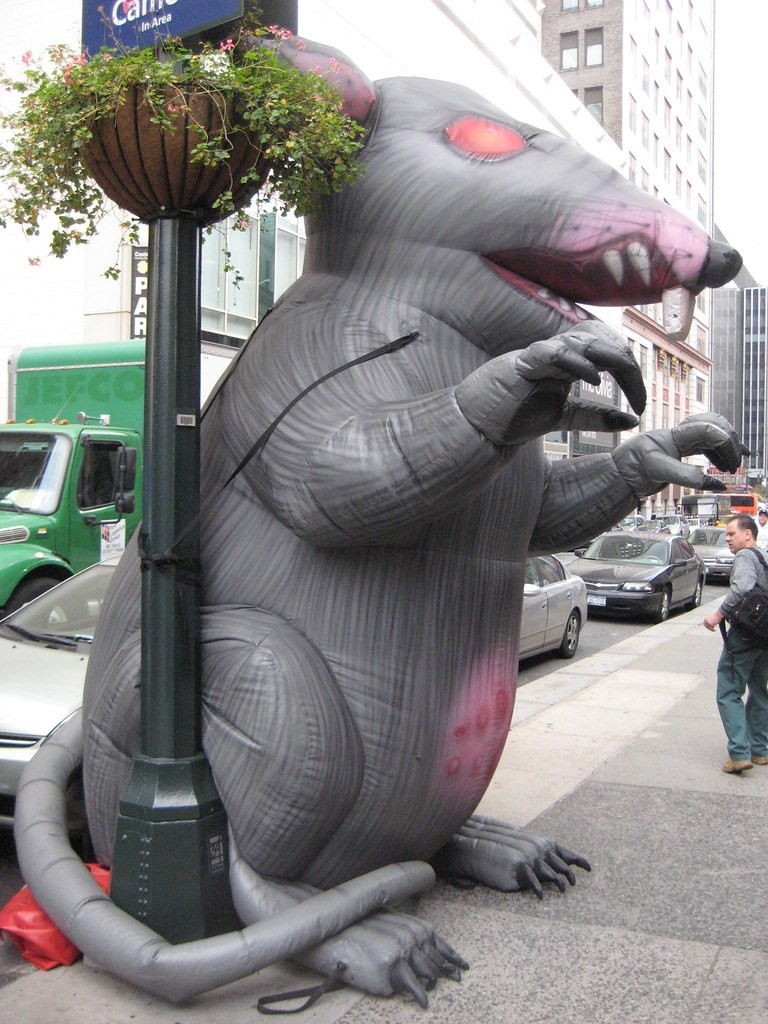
[720,547,768,654]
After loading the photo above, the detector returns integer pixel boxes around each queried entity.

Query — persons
[702,508,768,773]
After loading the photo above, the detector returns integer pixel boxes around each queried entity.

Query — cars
[568,514,736,623]
[517,553,589,662]
[0,553,119,863]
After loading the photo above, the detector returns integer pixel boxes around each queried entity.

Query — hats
[759,509,768,516]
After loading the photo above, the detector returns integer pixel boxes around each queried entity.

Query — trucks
[0,340,148,618]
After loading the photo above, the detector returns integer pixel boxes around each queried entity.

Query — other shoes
[751,755,768,765]
[722,758,752,773]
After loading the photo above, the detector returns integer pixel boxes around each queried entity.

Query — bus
[674,494,758,518]
